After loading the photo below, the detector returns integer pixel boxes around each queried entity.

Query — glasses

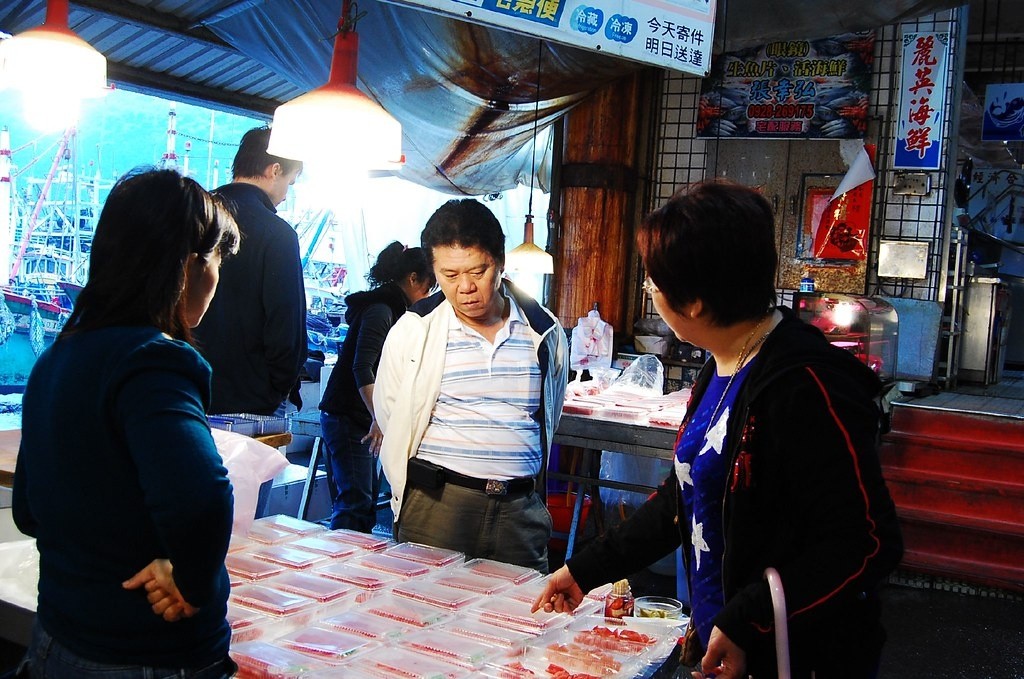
[642,280,659,294]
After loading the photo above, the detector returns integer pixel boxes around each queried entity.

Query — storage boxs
[261,464,332,521]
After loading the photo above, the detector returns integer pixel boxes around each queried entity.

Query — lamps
[0,0,107,92]
[504,214,555,275]
[266,0,405,170]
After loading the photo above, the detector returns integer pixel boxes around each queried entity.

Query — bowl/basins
[634,596,683,621]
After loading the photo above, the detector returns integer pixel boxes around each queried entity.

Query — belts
[443,467,533,497]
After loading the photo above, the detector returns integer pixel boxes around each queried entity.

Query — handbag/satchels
[679,626,706,668]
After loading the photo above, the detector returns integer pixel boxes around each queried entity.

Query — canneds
[604,591,634,624]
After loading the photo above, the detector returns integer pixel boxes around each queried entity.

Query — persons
[375,199,573,572]
[531,175,909,679]
[193,128,308,415]
[318,241,436,528]
[10,168,246,679]
